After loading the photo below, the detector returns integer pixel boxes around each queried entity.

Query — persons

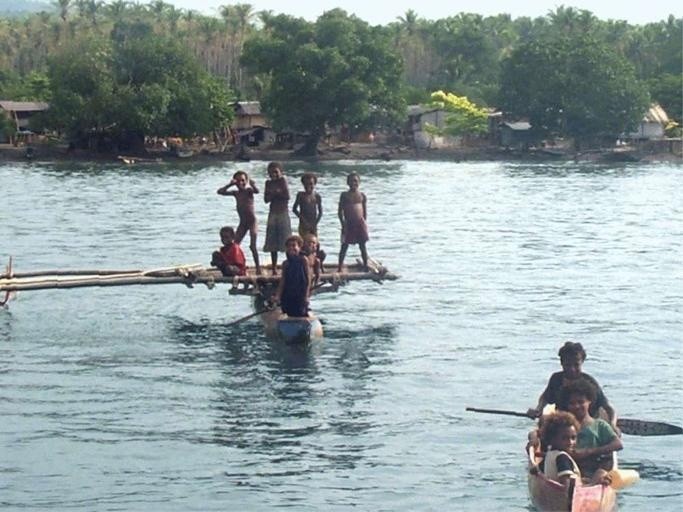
[211,161,369,317]
[526,341,623,487]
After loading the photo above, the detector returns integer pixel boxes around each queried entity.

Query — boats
[251,297,323,347]
[529,444,618,512]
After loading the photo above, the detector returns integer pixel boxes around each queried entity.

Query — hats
[282,232,304,248]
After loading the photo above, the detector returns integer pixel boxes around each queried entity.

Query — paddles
[464,406,683,438]
